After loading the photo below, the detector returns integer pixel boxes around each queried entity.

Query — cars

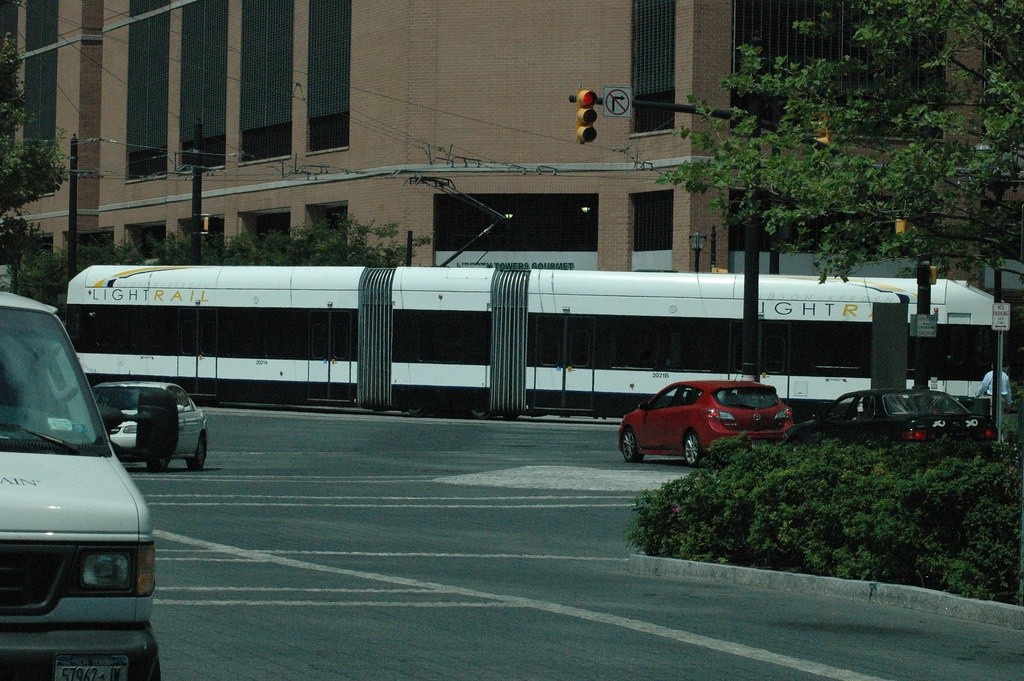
[784,388,1000,461]
[618,379,795,469]
[92,383,209,470]
[1,291,164,680]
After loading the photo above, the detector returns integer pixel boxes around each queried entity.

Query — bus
[63,265,1008,420]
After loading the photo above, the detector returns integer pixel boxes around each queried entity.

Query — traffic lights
[204,217,221,232]
[575,89,598,145]
[811,101,828,152]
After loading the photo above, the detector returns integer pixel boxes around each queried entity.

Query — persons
[975,362,1012,414]
